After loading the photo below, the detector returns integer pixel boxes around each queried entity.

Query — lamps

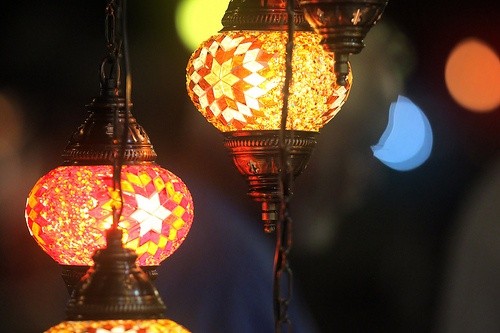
[300,1,386,86]
[185,0,353,238]
[41,229,193,333]
[25,0,196,296]
